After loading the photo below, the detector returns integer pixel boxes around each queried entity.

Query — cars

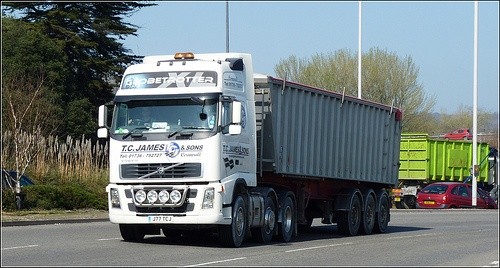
[416,182,498,210]
[445,130,472,141]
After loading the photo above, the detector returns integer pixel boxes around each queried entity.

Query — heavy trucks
[392,133,500,209]
[98,52,403,247]
